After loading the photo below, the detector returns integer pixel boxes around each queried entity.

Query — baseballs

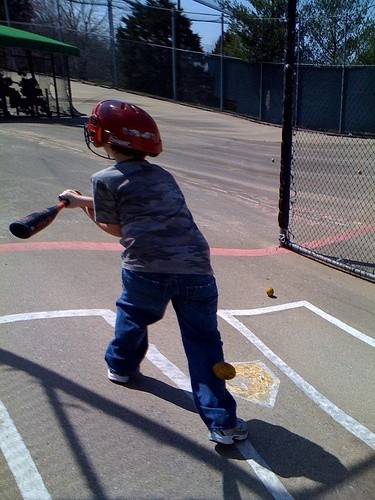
[212,361,236,380]
[266,287,274,297]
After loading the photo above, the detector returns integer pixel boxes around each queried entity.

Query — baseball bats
[9,190,82,239]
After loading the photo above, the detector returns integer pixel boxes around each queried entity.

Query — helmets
[83,100,162,160]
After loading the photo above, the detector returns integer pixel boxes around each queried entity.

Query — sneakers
[209,417,248,447]
[107,368,130,383]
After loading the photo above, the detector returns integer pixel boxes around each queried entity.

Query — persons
[55,98,253,449]
